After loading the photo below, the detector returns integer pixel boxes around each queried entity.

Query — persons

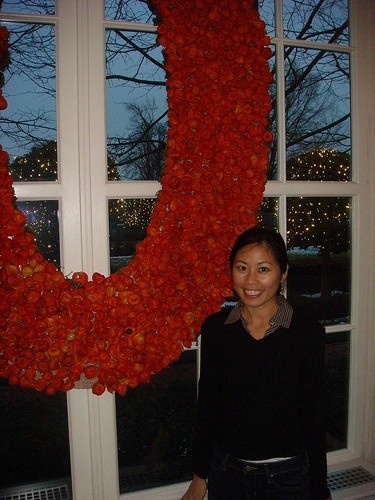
[181,227,332,500]
